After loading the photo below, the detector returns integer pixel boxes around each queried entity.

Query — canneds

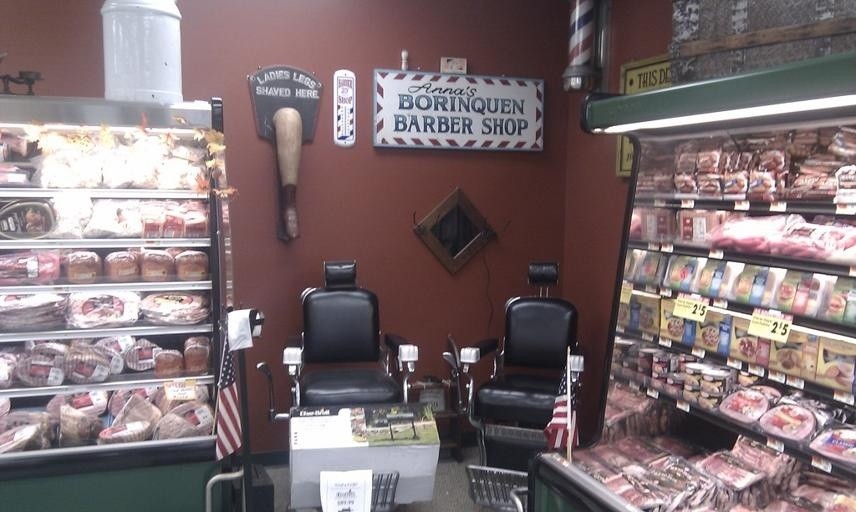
[609,336,733,412]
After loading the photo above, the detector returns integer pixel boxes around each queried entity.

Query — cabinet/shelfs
[528,53,855,509]
[0,91,270,510]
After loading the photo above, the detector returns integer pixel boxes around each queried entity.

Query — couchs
[460,262,585,466]
[282,258,419,411]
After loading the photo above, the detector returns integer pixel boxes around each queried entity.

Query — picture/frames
[412,184,499,277]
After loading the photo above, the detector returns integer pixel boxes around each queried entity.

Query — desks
[285,411,440,512]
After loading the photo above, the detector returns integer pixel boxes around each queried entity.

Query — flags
[543,364,580,449]
[215,336,244,462]
